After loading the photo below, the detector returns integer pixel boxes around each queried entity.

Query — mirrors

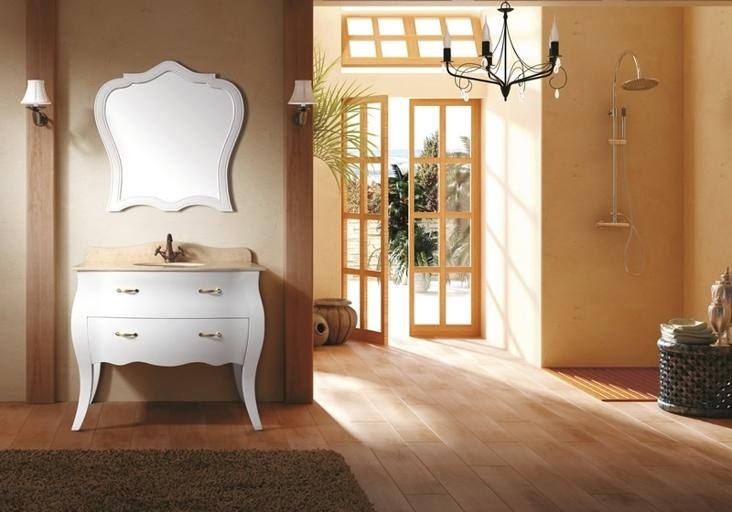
[91,58,247,215]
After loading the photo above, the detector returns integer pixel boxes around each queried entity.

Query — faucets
[155,233,185,260]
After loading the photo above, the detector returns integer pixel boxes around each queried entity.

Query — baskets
[657,338,732,418]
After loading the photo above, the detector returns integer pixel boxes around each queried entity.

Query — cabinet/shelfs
[71,240,268,433]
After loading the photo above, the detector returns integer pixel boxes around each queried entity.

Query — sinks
[135,260,202,270]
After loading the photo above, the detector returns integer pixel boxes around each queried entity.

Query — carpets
[546,366,661,402]
[0,448,377,512]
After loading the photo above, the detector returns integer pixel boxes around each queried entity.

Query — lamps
[21,79,53,127]
[441,1,569,102]
[287,79,319,127]
